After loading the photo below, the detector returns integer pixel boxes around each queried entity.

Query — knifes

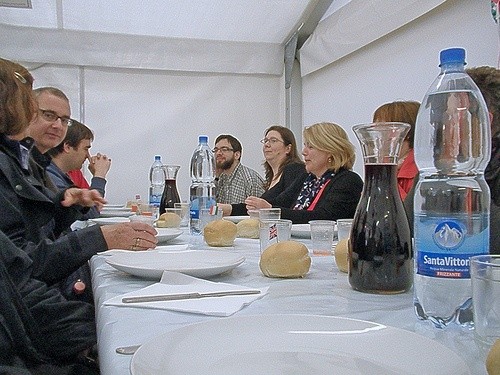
[122,290,261,303]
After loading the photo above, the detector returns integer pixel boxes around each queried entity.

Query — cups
[140,203,153,216]
[309,220,336,256]
[166,202,190,227]
[199,209,223,234]
[337,219,353,242]
[259,207,292,254]
[469,255,500,345]
[129,215,155,226]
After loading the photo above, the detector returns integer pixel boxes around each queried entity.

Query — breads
[204,219,237,247]
[259,241,311,278]
[485,337,500,375]
[334,238,349,273]
[236,218,261,239]
[157,212,181,228]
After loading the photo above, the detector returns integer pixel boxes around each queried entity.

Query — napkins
[102,272,270,317]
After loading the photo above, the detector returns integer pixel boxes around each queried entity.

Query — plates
[88,204,137,224]
[154,228,183,243]
[105,250,246,279]
[223,215,250,223]
[130,313,474,374]
[291,224,338,239]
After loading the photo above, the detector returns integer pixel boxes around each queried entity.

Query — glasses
[260,137,288,146]
[38,108,74,126]
[212,147,234,152]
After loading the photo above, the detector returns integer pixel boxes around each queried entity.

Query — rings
[135,243,139,247]
[132,246,136,251]
[136,238,140,243]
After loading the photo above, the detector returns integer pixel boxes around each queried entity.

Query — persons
[18,87,112,220]
[0,56,159,303]
[217,121,364,224]
[402,66,500,258]
[211,133,267,205]
[0,232,101,375]
[260,124,306,201]
[372,100,421,203]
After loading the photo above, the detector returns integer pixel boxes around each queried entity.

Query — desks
[85,203,500,375]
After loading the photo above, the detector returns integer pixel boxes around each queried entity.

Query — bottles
[348,120,411,294]
[159,165,181,216]
[412,48,491,329]
[147,155,166,222]
[189,136,217,237]
